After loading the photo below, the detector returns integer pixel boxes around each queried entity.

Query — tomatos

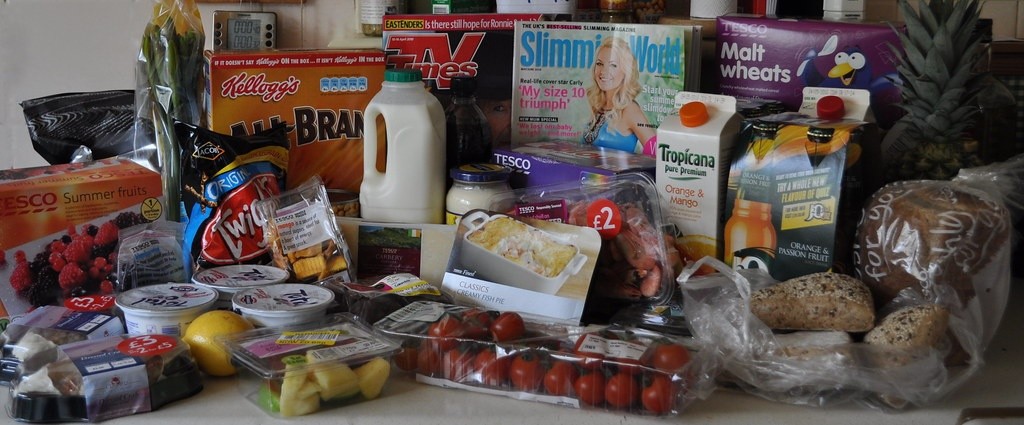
[394,309,688,415]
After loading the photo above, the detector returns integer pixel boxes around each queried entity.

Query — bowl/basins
[192,264,290,312]
[114,282,220,337]
[462,211,588,295]
[231,283,335,328]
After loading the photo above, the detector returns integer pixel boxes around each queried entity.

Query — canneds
[446,162,514,227]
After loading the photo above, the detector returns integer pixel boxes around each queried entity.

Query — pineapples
[281,356,389,415]
[885,0,999,181]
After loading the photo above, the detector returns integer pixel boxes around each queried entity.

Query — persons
[580,37,656,154]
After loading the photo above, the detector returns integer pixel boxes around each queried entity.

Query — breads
[745,182,1010,411]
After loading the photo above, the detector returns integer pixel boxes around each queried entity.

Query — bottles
[974,17,1022,165]
[359,69,447,223]
[724,170,777,274]
[445,76,492,190]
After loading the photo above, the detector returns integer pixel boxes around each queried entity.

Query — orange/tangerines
[674,235,716,277]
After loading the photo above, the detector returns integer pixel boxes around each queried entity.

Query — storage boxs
[441,210,603,328]
[204,46,387,190]
[0,157,163,263]
[724,86,884,280]
[489,137,656,190]
[717,16,907,110]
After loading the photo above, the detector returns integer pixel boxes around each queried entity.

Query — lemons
[184,310,255,376]
